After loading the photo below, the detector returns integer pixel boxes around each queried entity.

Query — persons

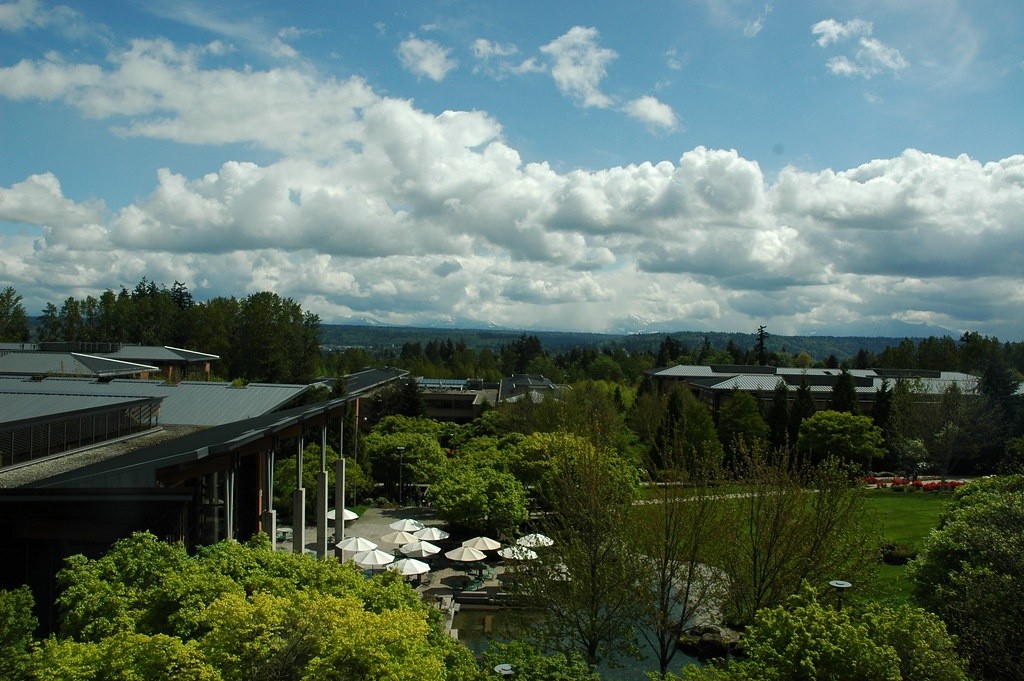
[905,464,911,480]
[912,467,918,483]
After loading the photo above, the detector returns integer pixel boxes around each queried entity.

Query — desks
[277,528,292,539]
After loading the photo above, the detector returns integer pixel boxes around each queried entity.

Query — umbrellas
[326,508,554,582]
